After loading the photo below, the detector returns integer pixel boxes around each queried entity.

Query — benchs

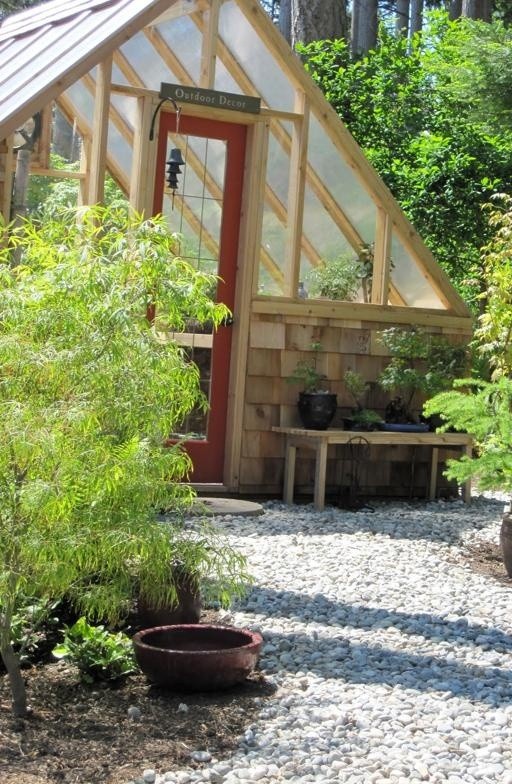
[274,426,475,503]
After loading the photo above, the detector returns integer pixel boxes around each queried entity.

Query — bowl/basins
[134,625,264,693]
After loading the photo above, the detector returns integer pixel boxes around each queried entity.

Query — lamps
[150,96,183,191]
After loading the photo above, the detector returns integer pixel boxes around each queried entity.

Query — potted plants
[294,326,469,435]
